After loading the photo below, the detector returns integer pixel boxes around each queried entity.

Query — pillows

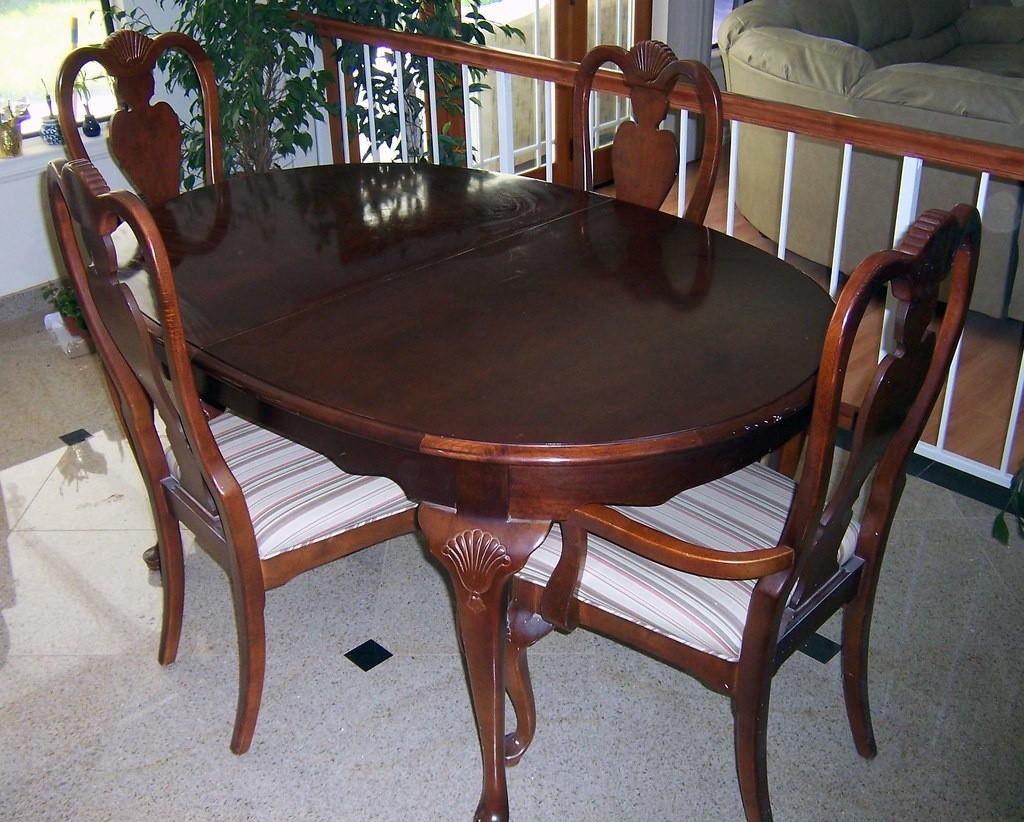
[955,6,1024,44]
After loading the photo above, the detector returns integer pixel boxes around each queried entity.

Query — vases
[0,122,25,158]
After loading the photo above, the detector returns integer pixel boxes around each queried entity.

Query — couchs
[728,0,1024,322]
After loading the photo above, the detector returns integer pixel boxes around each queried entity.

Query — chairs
[413,204,983,822]
[572,39,724,221]
[44,160,420,755]
[55,28,228,426]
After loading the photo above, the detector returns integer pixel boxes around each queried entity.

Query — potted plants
[73,68,105,138]
[39,77,66,146]
[42,283,85,336]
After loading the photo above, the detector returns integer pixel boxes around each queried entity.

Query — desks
[110,162,836,822]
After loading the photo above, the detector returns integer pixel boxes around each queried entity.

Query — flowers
[0,95,31,127]
